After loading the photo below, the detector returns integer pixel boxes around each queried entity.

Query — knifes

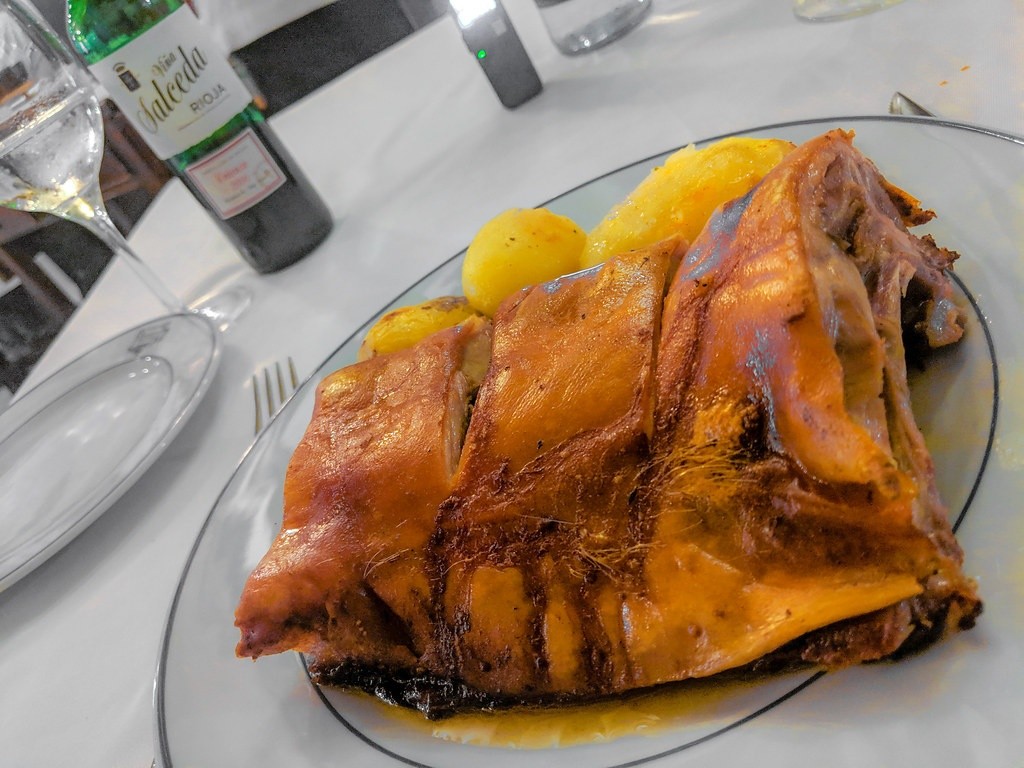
[889,90,937,117]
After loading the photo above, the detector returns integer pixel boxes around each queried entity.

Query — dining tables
[0,2,1024,768]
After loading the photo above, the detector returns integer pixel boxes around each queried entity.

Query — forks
[252,357,300,435]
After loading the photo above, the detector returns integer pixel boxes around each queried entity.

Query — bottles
[64,0,334,274]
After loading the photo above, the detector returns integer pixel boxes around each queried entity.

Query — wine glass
[0,0,248,332]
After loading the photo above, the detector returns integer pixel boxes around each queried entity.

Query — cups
[533,0,655,58]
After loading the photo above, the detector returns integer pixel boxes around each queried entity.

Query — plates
[152,112,1024,768]
[0,308,224,595]
